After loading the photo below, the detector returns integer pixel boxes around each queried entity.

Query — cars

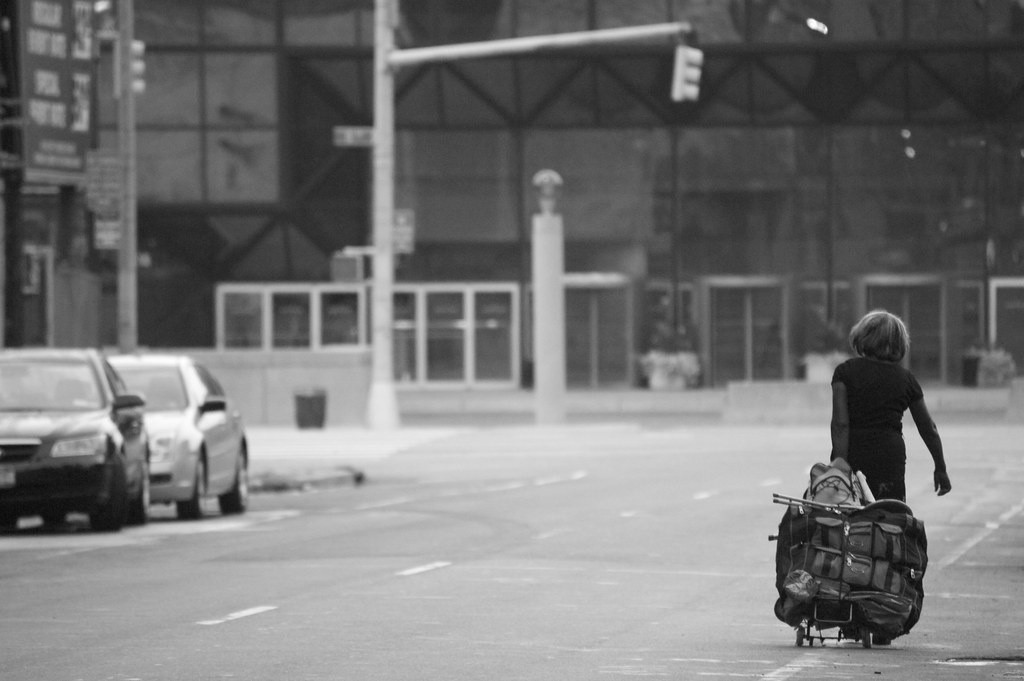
[64,353,253,523]
[0,345,153,529]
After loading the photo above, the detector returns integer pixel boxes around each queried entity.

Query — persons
[830,309,951,644]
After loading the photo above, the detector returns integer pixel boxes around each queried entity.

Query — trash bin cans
[296,391,326,429]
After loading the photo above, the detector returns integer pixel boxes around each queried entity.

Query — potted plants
[798,350,851,384]
[962,345,1018,389]
[635,347,703,390]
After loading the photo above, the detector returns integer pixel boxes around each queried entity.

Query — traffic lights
[667,46,706,102]
[109,34,147,99]
